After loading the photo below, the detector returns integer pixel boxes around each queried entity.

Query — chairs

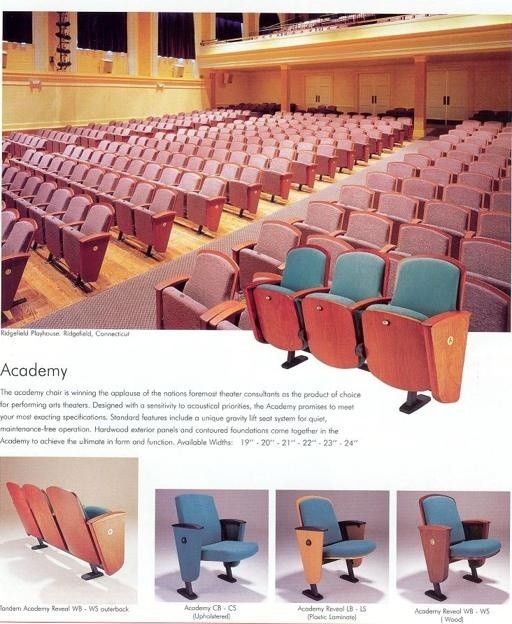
[6,482,44,550]
[46,486,126,579]
[295,495,377,601]
[417,494,503,603]
[23,483,67,553]
[171,494,259,600]
